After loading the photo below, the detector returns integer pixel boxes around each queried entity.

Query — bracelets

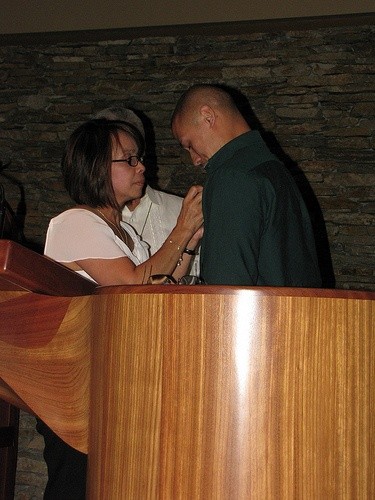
[166,232,182,267]
[184,248,197,256]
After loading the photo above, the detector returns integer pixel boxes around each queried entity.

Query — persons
[88,106,200,283]
[171,83,324,287]
[34,118,203,500]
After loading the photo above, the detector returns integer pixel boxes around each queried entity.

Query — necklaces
[94,206,129,248]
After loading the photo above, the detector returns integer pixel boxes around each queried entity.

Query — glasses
[112,155,150,167]
